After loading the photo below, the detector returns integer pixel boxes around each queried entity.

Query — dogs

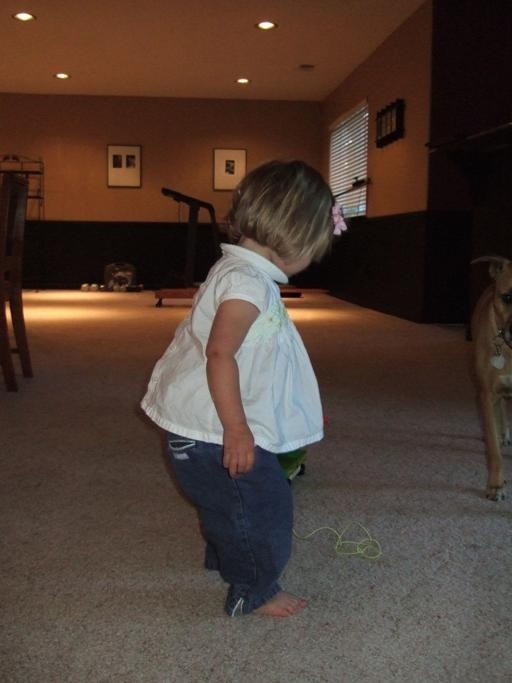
[468,256,512,503]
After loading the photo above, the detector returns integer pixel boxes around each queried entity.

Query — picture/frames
[106,144,143,188]
[213,147,248,192]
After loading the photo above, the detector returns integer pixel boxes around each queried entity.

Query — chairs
[0,172,34,392]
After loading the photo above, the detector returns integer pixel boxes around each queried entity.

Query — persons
[137,155,348,617]
